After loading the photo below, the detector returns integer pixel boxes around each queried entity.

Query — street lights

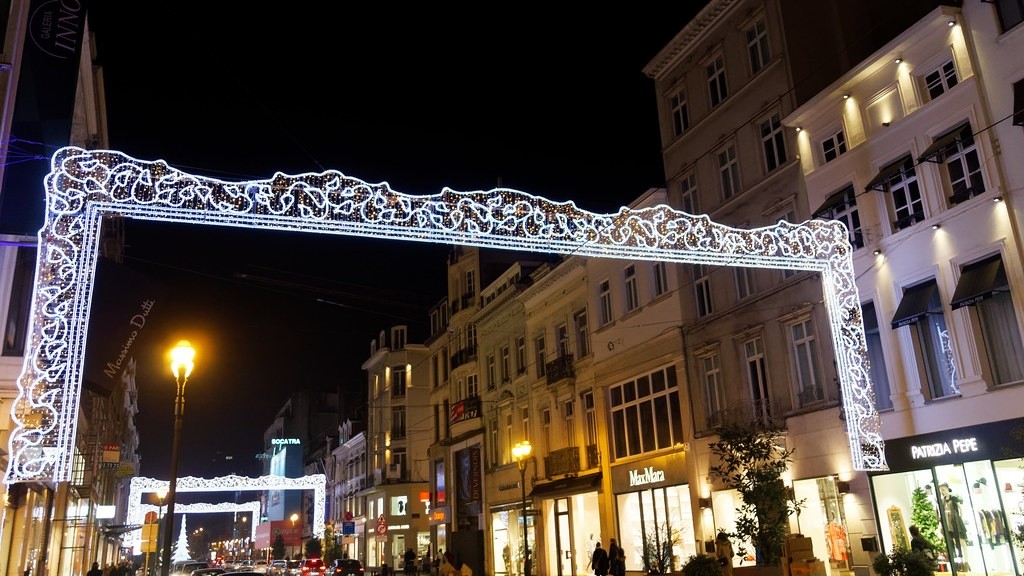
[290,513,299,560]
[512,440,532,575]
[161,338,196,575]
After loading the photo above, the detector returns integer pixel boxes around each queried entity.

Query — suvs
[299,559,325,576]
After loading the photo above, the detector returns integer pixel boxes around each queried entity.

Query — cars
[280,560,301,576]
[266,560,285,576]
[324,559,365,576]
[170,558,267,576]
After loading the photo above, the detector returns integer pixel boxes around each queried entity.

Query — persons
[592,542,610,575]
[938,484,968,559]
[715,533,734,576]
[605,538,619,575]
[368,542,450,576]
[909,529,938,576]
[612,548,626,576]
